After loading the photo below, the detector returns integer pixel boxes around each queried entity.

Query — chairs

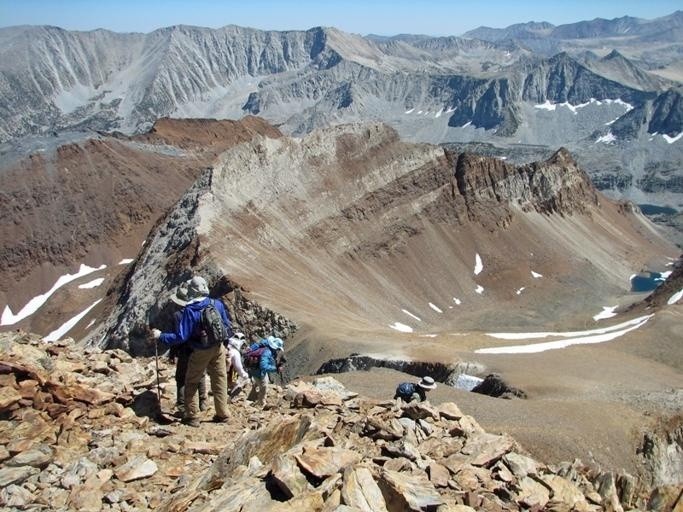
[213,415,229,422]
[181,418,200,427]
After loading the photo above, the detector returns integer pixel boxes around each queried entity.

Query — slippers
[198,299,228,346]
[243,347,266,367]
[396,382,416,402]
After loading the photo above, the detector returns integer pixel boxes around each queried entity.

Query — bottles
[267,336,285,353]
[418,376,437,390]
[170,276,209,307]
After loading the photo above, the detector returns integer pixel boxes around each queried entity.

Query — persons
[150,275,234,428]
[241,336,286,412]
[167,287,208,413]
[392,376,437,405]
[207,331,250,404]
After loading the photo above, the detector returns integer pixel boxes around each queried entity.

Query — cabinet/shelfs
[201,330,208,347]
[250,355,258,362]
